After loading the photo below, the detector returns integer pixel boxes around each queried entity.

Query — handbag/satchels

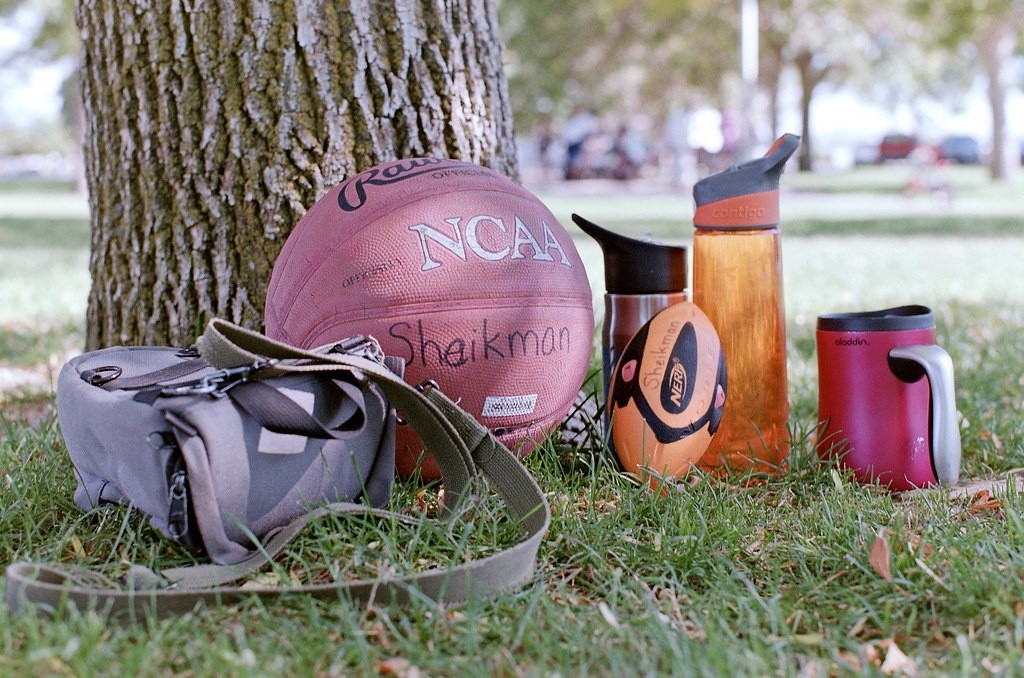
[54,342,405,557]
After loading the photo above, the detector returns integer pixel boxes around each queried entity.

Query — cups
[814,304,963,493]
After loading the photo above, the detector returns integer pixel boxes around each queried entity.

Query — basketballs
[264,157,595,482]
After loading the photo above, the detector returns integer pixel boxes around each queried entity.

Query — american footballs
[604,301,726,497]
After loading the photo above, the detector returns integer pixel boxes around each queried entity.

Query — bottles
[574,215,688,472]
[692,133,800,485]
